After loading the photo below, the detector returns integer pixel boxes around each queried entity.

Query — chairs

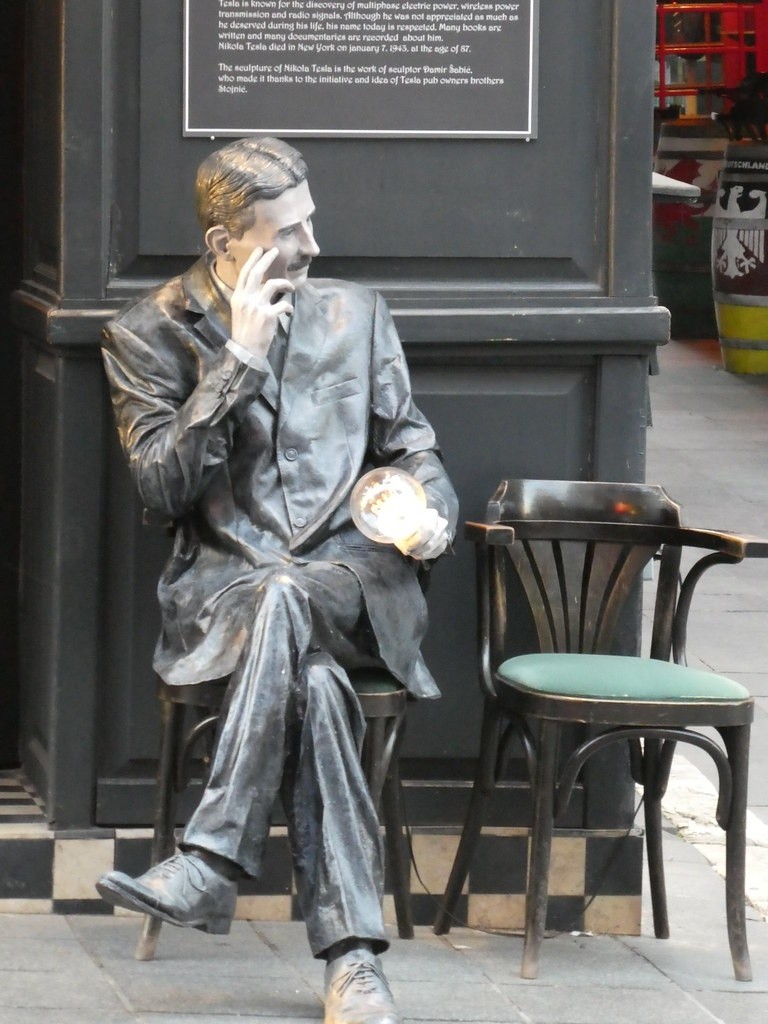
[134,504,440,953]
[437,479,768,982]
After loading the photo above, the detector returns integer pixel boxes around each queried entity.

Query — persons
[93,135,461,1024]
